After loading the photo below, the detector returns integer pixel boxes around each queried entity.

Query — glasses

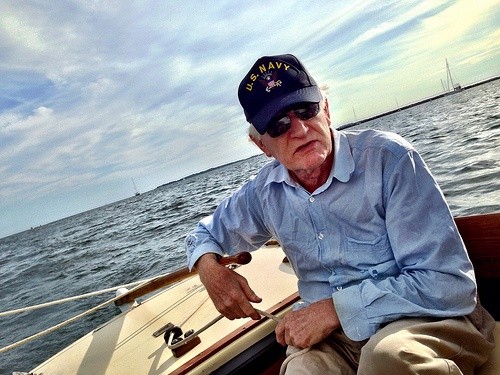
[266,102,320,137]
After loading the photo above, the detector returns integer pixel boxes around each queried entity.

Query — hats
[238,53,321,136]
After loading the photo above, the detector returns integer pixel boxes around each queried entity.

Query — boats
[1,211,499,375]
